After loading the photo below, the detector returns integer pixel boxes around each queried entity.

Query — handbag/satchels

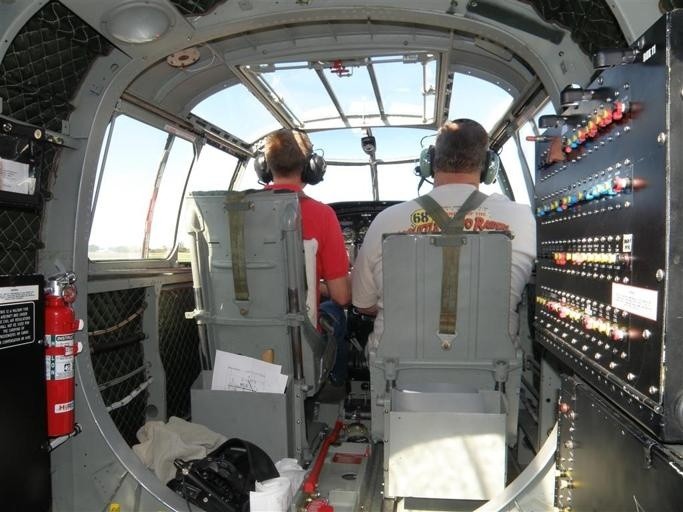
[167,437,280,511]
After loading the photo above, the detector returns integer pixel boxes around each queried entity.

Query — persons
[256,127,352,380]
[349,117,538,370]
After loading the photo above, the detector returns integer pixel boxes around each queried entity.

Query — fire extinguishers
[45,270,83,436]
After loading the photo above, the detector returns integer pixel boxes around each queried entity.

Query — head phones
[414,144,500,184]
[253,129,326,185]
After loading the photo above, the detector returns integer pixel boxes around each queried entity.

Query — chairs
[366,232,524,451]
[184,189,336,399]
